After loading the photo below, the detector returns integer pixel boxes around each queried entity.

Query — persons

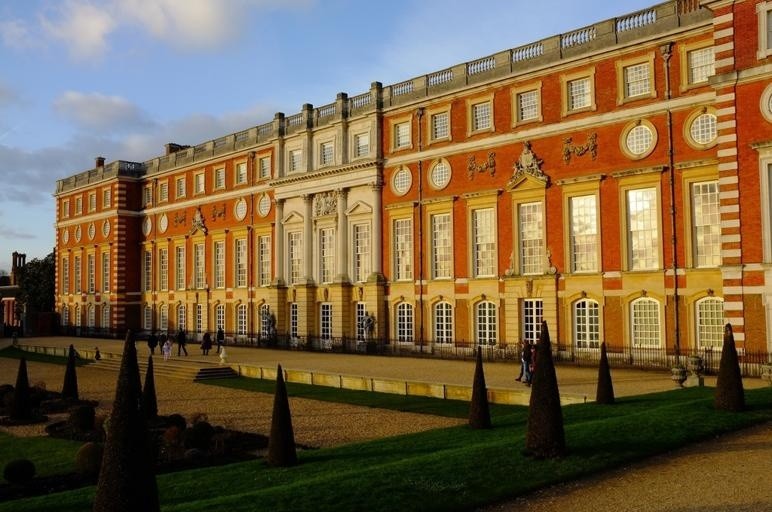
[145,326,226,362]
[261,311,274,333]
[516,338,536,386]
[93,346,101,363]
[360,310,374,338]
